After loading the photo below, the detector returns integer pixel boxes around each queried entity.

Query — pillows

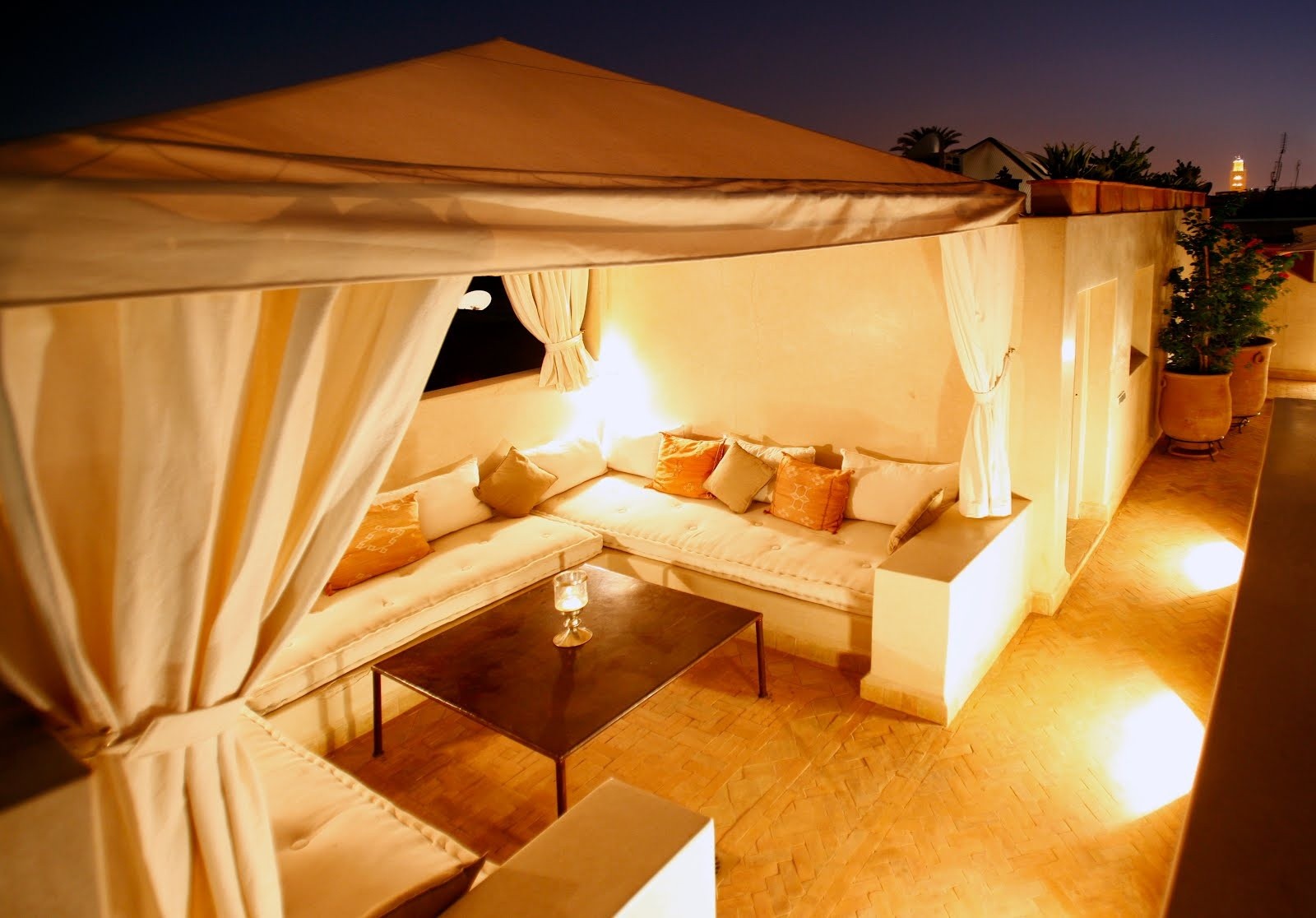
[322,421,960,595]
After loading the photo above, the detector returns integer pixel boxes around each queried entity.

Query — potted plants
[1024,134,1210,216]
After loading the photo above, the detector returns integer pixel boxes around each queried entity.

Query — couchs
[237,509,604,754]
[0,710,493,918]
[533,461,939,674]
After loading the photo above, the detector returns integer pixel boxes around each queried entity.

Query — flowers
[1147,204,1300,376]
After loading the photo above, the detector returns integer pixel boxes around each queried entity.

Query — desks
[371,564,765,816]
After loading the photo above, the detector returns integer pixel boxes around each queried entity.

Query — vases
[1156,366,1235,457]
[1202,333,1277,421]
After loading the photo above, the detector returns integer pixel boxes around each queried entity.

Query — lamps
[553,570,593,647]
